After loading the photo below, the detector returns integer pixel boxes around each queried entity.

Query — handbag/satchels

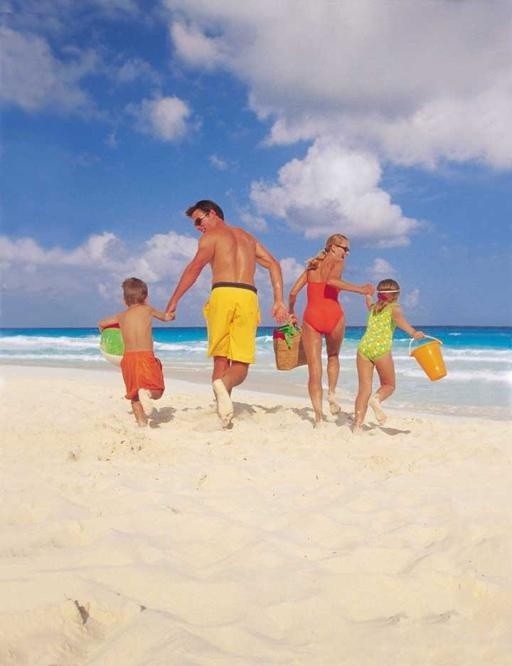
[273,329,307,370]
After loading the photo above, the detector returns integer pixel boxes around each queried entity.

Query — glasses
[335,244,349,252]
[194,212,209,226]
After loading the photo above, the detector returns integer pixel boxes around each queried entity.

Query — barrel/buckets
[408,335,447,382]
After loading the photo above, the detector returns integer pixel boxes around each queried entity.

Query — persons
[165,200,289,428]
[98,277,175,427]
[289,234,374,429]
[352,279,425,433]
